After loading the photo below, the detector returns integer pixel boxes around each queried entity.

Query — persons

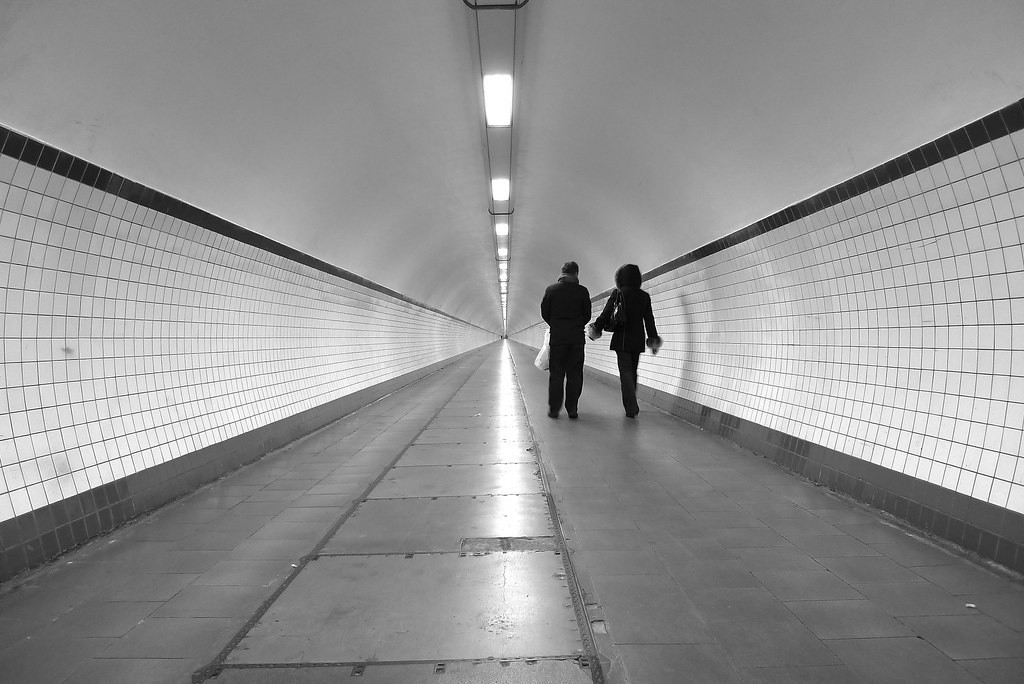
[541,260,592,419]
[587,263,663,418]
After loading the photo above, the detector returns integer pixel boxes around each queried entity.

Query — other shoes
[568,412,579,418]
[548,410,559,418]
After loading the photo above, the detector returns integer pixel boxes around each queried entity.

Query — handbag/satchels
[534,328,550,371]
[604,289,624,332]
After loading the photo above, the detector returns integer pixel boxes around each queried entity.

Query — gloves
[646,337,664,355]
[587,323,603,342]
